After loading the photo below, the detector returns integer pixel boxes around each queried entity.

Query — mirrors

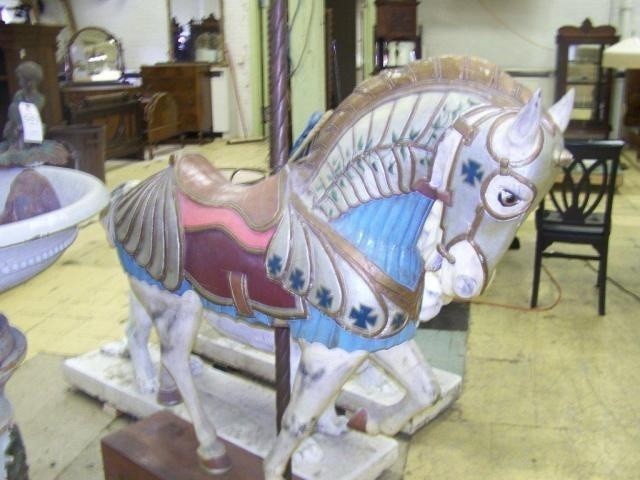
[28,0,79,80]
[166,1,230,69]
[184,13,222,63]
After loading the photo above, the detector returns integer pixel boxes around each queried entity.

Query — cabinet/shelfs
[372,0,425,78]
[553,16,622,143]
[0,19,215,165]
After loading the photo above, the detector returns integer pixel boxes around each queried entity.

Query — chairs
[532,138,622,315]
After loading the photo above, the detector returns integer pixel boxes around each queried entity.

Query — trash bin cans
[48,125,106,185]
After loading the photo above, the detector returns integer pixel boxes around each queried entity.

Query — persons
[1,60,68,165]
